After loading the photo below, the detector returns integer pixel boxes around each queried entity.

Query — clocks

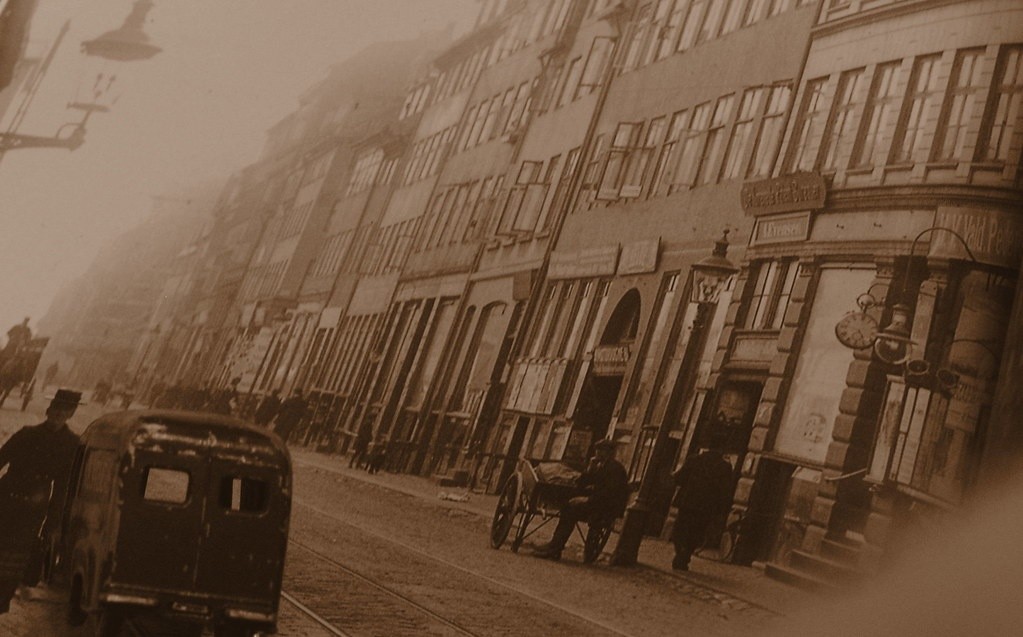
[836,312,880,350]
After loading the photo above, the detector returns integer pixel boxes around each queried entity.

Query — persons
[658,440,732,570]
[148,375,307,444]
[1,386,86,614]
[349,412,377,468]
[534,440,629,560]
[0,317,32,366]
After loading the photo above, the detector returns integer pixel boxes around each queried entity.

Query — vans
[43,406,294,637]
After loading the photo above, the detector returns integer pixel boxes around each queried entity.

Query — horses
[0,356,24,409]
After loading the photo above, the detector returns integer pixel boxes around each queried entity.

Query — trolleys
[489,454,630,565]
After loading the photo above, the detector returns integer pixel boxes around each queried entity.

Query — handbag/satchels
[24,536,47,588]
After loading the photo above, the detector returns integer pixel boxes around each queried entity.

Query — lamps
[0,0,171,151]
[873,228,979,365]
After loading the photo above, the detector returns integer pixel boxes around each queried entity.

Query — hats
[594,438,617,450]
[46,387,87,406]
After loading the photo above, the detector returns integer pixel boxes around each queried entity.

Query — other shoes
[529,542,563,561]
